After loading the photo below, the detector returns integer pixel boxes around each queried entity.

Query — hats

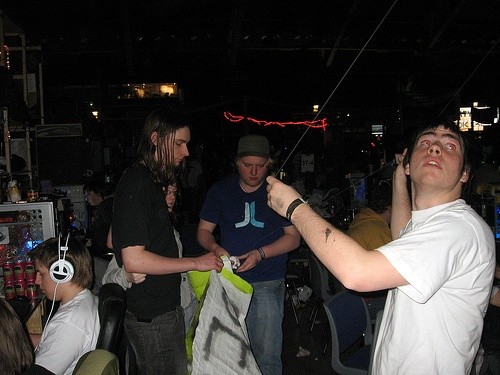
[238,135,269,157]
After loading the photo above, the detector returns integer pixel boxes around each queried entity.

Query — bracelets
[256,247,265,260]
[286,196,309,222]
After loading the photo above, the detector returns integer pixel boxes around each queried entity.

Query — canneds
[4,256,36,299]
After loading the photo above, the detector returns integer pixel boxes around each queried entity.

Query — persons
[329,183,393,299]
[25,232,101,375]
[469,262,500,375]
[196,134,301,375]
[266,119,496,375]
[83,109,224,375]
[0,297,34,375]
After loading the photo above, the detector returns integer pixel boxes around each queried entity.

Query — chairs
[70,282,127,375]
[287,254,374,375]
